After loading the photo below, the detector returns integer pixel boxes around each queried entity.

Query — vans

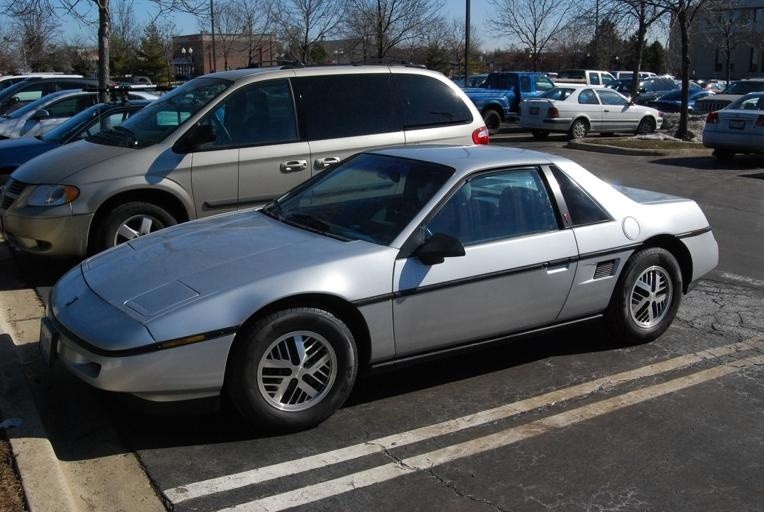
[1,64,488,257]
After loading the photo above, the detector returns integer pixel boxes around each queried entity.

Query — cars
[44,144,721,430]
[553,70,763,154]
[2,72,170,185]
[517,86,663,138]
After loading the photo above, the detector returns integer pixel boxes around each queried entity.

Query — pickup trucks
[450,70,555,134]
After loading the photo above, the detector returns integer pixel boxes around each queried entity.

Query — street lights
[334,46,347,63]
[179,44,195,77]
